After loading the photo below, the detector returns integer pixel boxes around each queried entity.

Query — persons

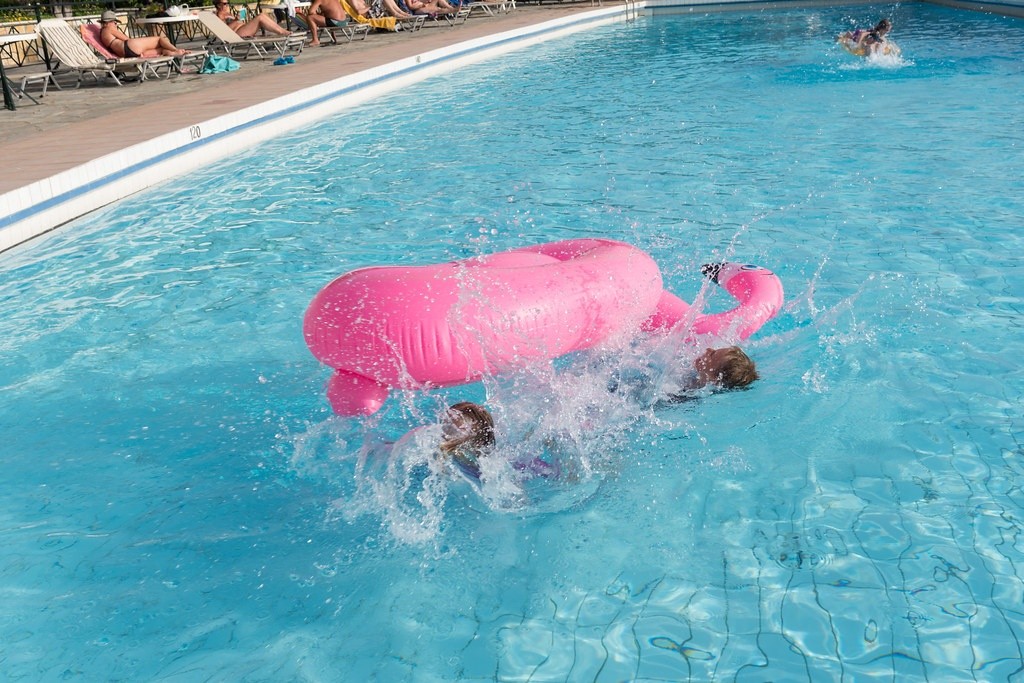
[844,18,893,47]
[306,0,347,46]
[99,10,192,58]
[347,0,411,18]
[442,402,496,456]
[156,6,169,17]
[212,0,293,36]
[404,0,462,15]
[666,345,758,404]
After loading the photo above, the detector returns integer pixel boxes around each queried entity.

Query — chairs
[1,1,522,110]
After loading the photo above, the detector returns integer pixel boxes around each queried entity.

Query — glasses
[219,2,230,4]
[111,21,117,25]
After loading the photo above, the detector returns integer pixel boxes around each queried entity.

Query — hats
[97,11,122,23]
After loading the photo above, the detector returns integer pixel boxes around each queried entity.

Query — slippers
[177,68,191,73]
[284,56,295,62]
[273,57,288,65]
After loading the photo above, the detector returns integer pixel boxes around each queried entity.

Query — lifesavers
[303,237,786,419]
